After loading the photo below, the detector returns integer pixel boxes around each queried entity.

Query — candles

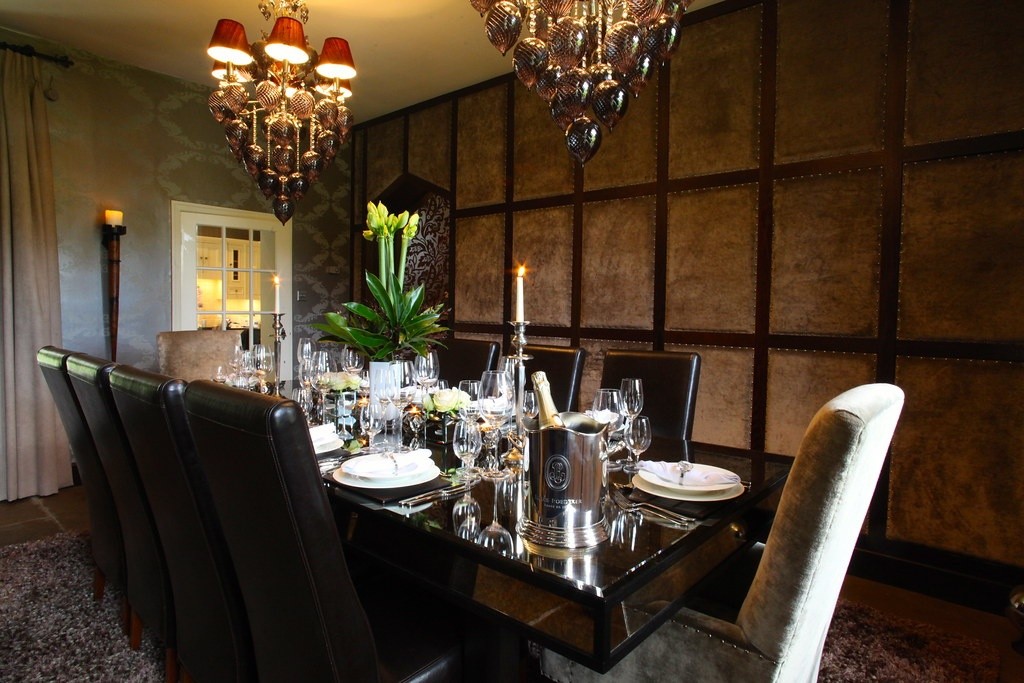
[273,276,281,312]
[517,266,527,322]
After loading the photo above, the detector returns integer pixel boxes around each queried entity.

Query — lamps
[202,0,357,224]
[103,209,126,363]
[472,0,698,166]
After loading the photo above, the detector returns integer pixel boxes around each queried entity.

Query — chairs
[35,329,700,683]
[540,383,907,683]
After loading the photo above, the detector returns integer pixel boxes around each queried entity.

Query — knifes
[398,479,476,506]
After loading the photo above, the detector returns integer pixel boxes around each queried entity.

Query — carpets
[0,530,963,682]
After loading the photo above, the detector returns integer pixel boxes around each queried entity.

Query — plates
[632,473,745,502]
[315,438,345,454]
[639,463,741,494]
[342,452,435,482]
[333,466,440,489]
[313,432,339,448]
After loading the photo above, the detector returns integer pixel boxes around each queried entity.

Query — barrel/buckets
[515,411,613,549]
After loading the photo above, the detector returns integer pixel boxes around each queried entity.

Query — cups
[202,319,206,327]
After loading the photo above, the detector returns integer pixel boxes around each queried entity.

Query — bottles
[531,369,566,429]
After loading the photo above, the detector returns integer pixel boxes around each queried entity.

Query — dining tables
[243,382,795,683]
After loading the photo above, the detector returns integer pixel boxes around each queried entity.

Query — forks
[609,487,696,527]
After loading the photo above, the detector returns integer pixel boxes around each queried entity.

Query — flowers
[308,200,452,361]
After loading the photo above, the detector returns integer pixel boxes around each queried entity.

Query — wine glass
[451,482,645,560]
[212,337,652,483]
